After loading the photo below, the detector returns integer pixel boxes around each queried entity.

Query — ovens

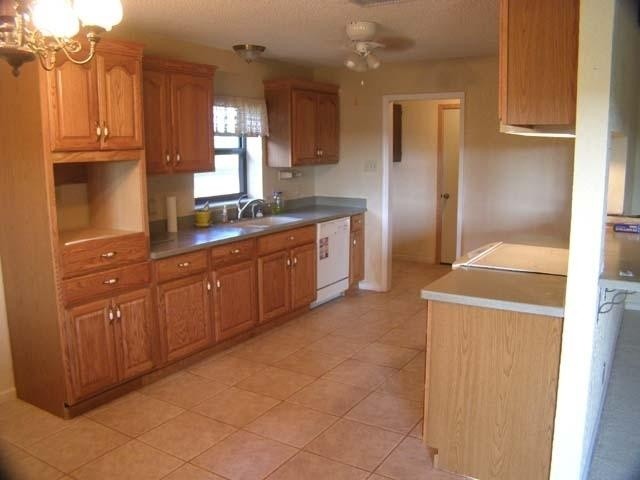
[310,218,350,311]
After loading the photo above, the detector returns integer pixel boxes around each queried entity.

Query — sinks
[231,215,303,229]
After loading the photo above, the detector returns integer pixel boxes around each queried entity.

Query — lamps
[0,0,130,79]
[232,42,266,64]
[338,17,387,76]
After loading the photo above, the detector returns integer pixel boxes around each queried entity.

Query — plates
[192,221,213,228]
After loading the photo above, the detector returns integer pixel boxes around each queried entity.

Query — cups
[195,210,210,222]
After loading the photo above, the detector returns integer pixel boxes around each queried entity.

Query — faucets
[251,202,264,218]
[237,194,263,221]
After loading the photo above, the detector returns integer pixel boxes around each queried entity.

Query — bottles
[272,191,285,213]
[223,205,228,222]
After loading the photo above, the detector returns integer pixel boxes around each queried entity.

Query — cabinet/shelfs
[0,156,158,418]
[259,225,319,333]
[149,53,220,174]
[350,212,369,292]
[260,79,339,168]
[416,266,568,480]
[495,1,574,140]
[155,234,260,374]
[0,37,150,156]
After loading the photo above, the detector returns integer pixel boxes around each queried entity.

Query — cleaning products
[276,192,283,214]
[270,192,278,214]
